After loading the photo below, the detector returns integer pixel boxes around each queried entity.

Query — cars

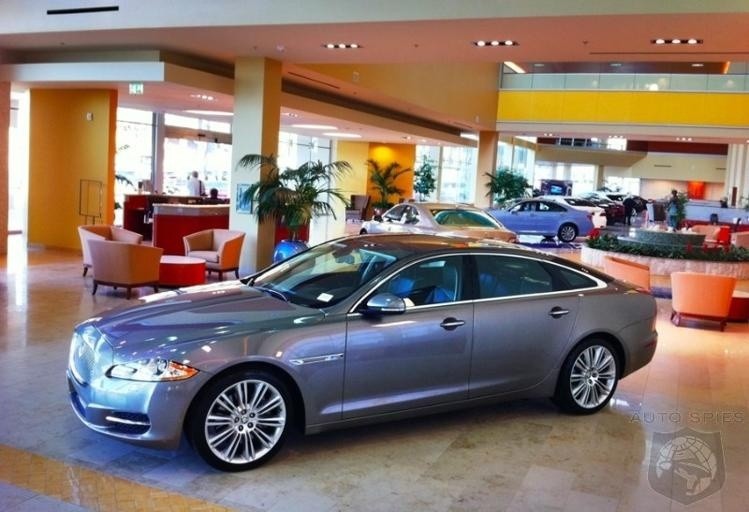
[66,203,657,472]
[489,192,648,242]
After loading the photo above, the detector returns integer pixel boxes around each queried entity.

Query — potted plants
[364,157,412,217]
[234,150,355,265]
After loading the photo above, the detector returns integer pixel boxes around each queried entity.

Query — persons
[622,194,637,226]
[186,171,206,200]
[667,189,682,230]
[209,188,219,200]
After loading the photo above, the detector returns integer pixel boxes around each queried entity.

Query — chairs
[690,223,748,252]
[378,248,458,306]
[77,222,246,300]
[344,194,371,224]
[604,255,737,335]
